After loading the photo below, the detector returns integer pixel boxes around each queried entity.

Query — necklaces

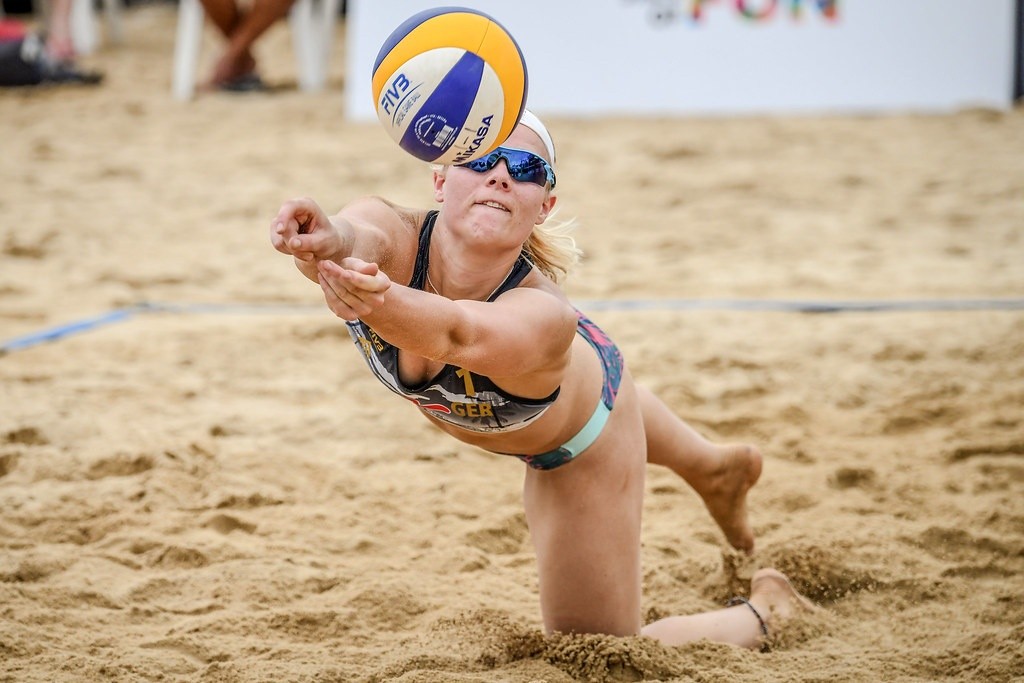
[426,271,439,294]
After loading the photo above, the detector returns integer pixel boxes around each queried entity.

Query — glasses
[453,145,556,190]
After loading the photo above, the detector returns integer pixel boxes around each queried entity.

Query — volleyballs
[372,6,529,165]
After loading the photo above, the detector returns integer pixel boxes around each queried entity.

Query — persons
[43,0,78,63]
[266,109,829,655]
[198,0,296,94]
